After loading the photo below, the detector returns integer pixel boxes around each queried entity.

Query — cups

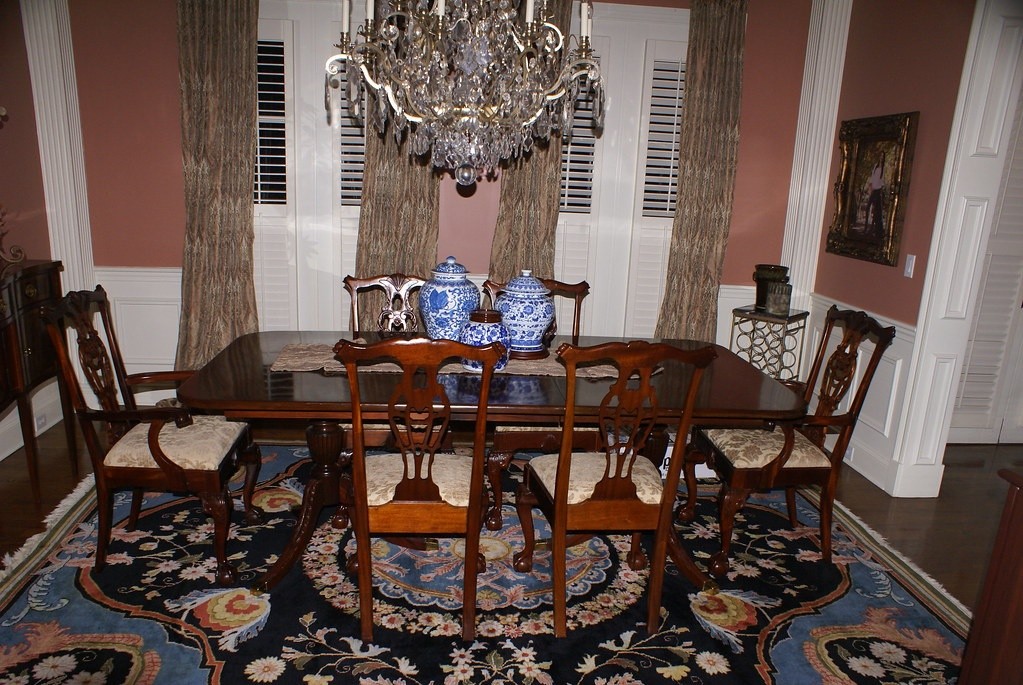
[764,283,792,315]
[753,263,790,310]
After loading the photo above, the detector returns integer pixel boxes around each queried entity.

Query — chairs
[482,278,602,532]
[514,338,720,640]
[334,337,507,646]
[343,272,426,332]
[677,304,896,577]
[41,283,263,588]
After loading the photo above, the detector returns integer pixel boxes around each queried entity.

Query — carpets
[0,442,973,685]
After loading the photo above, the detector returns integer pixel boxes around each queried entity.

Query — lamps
[325,0,605,186]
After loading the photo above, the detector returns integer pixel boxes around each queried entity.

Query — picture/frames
[826,110,920,266]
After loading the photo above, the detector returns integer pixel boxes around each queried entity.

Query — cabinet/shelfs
[0,245,79,480]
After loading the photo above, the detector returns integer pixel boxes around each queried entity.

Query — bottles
[460,309,510,372]
[495,269,554,353]
[419,256,481,342]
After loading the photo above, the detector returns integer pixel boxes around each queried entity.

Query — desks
[177,330,805,593]
[729,303,809,381]
